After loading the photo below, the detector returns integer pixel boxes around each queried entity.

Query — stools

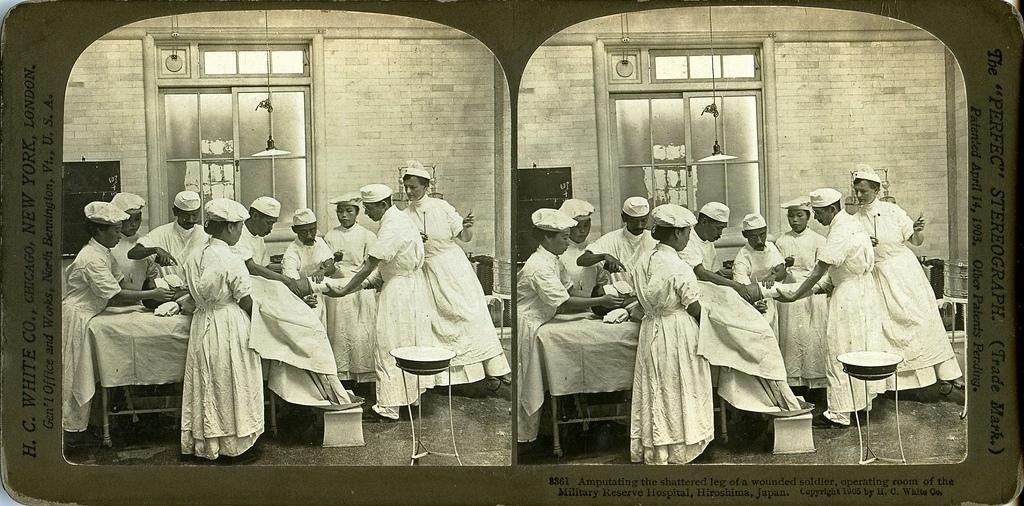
[311,389,367,449]
[764,395,817,455]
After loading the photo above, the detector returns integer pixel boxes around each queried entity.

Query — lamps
[696,4,738,163]
[250,10,291,157]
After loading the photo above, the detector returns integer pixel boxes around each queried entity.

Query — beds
[72,299,196,450]
[302,286,378,384]
[774,292,831,390]
[518,311,730,457]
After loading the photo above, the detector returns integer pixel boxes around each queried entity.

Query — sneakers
[812,413,851,429]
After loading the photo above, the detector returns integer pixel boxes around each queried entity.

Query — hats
[290,208,317,226]
[850,171,883,192]
[742,212,767,233]
[530,207,580,234]
[649,203,699,230]
[558,198,596,223]
[327,194,364,209]
[358,183,395,204]
[402,167,431,181]
[173,190,282,224]
[780,195,812,211]
[82,191,146,228]
[698,201,731,223]
[809,188,843,209]
[620,195,651,219]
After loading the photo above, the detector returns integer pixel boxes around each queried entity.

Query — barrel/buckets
[491,257,512,293]
[943,260,969,298]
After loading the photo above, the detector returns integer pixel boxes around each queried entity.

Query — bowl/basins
[835,351,903,380]
[388,346,457,374]
